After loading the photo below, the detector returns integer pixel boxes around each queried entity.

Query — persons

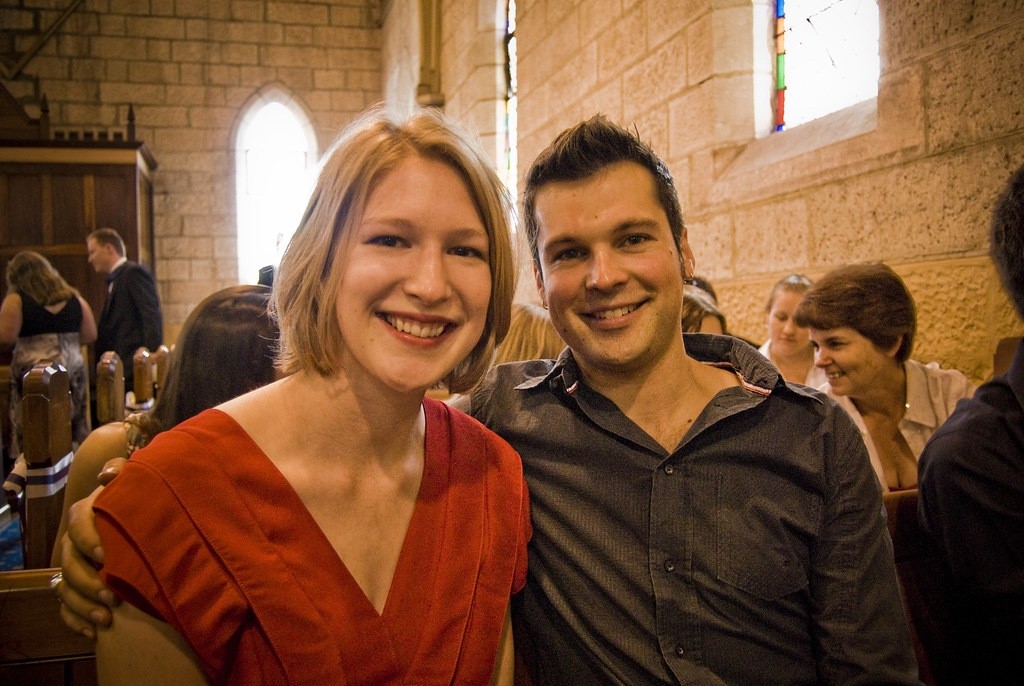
[0,229,285,568]
[49,116,921,686]
[93,117,532,686]
[682,260,985,494]
[912,162,1024,686]
[450,303,568,398]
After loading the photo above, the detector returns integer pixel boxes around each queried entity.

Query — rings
[51,573,63,590]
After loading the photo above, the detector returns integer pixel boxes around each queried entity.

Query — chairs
[94,350,125,425]
[133,346,177,406]
[20,362,73,573]
